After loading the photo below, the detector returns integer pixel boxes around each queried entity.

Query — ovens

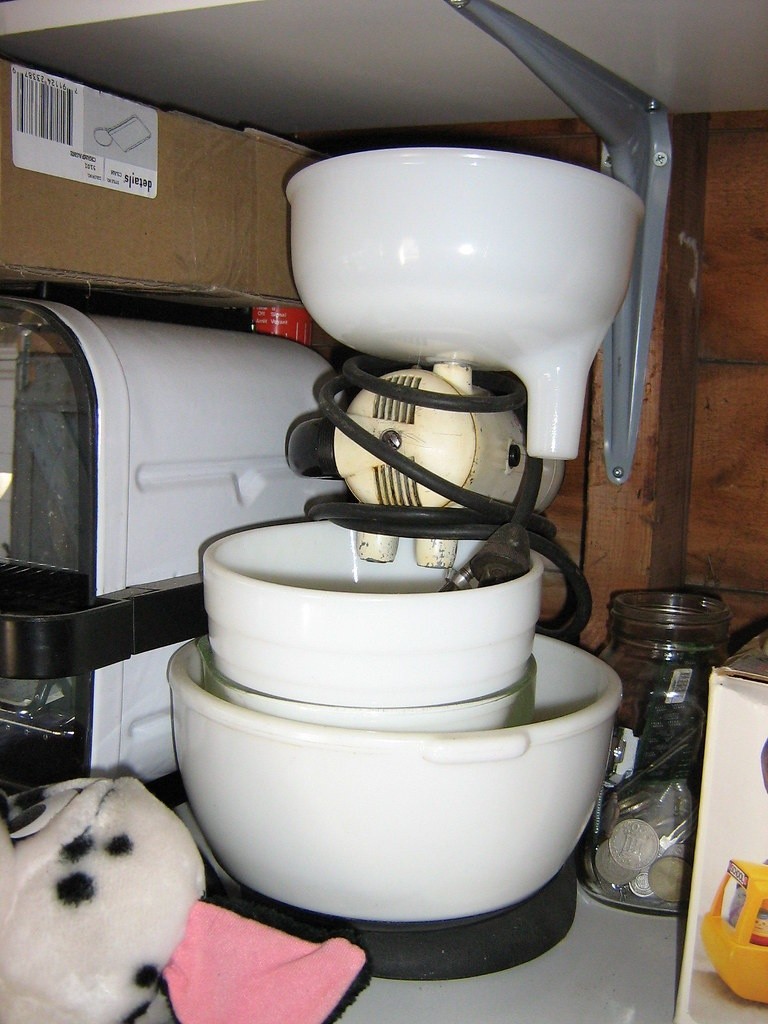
[0,289,347,783]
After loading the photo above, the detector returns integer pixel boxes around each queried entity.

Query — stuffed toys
[0,777,373,1024]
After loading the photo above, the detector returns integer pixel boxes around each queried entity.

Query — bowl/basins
[203,520,544,707]
[195,635,537,730]
[167,633,624,919]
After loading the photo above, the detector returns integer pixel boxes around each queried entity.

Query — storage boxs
[0,53,333,308]
[671,629,768,1024]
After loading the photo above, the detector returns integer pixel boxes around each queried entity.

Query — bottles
[576,590,733,912]
